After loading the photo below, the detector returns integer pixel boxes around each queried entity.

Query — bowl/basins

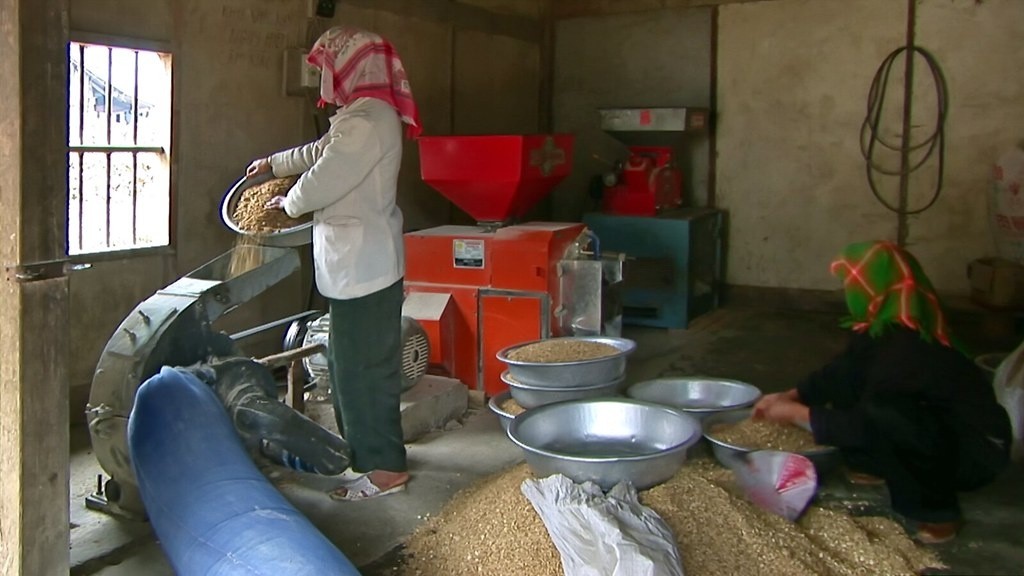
[488,334,837,493]
[220,172,314,249]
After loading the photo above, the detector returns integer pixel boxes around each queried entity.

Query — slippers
[328,475,407,500]
[337,466,372,482]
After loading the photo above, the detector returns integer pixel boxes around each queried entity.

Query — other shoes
[909,523,956,546]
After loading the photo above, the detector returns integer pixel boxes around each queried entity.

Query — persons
[246,26,423,502]
[752,241,1013,543]
